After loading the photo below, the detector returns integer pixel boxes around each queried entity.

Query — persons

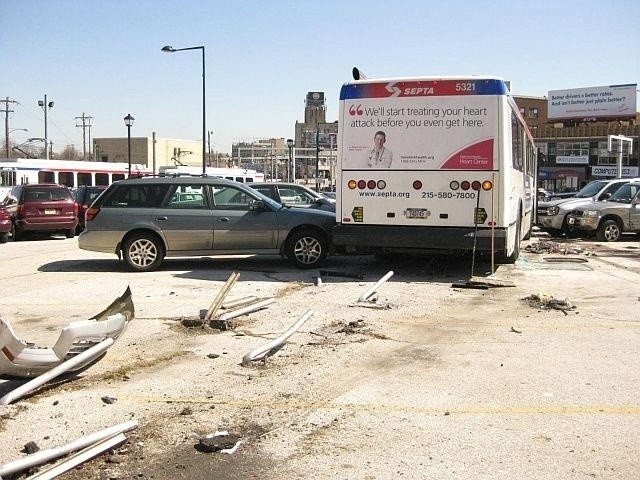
[366,131,394,168]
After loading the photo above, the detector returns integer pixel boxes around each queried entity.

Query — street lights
[160,45,209,176]
[123,113,135,199]
[5,126,30,159]
[286,138,294,183]
[316,120,339,193]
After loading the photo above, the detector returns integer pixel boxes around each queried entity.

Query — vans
[71,184,108,234]
[77,176,336,271]
[3,182,79,240]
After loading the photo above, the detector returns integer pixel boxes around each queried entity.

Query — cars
[1,204,11,244]
[170,192,205,205]
[566,181,640,242]
[242,183,336,226]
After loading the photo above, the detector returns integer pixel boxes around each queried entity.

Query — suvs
[537,179,617,239]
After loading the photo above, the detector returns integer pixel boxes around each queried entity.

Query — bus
[0,162,160,209]
[161,165,264,183]
[334,77,539,264]
